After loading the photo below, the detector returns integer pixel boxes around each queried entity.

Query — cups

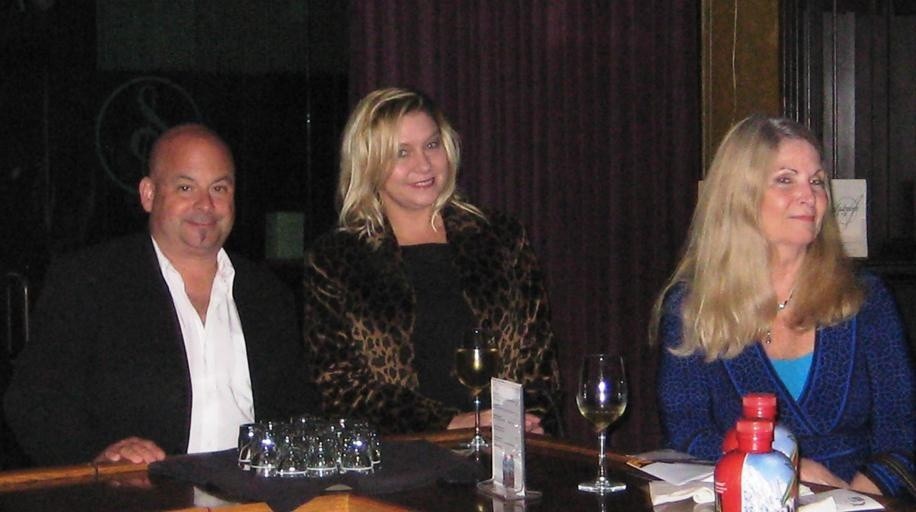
[236,416,382,481]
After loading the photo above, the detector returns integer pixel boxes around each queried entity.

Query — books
[625,447,719,485]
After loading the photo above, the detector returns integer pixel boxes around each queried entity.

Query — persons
[300,86,569,441]
[0,120,303,468]
[647,110,915,499]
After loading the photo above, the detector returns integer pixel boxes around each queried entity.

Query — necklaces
[763,282,796,343]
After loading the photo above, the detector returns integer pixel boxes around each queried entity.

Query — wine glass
[453,327,501,459]
[576,353,628,493]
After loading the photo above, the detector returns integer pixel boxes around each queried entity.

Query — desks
[0,427,916,511]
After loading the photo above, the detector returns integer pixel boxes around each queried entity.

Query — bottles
[715,393,799,512]
